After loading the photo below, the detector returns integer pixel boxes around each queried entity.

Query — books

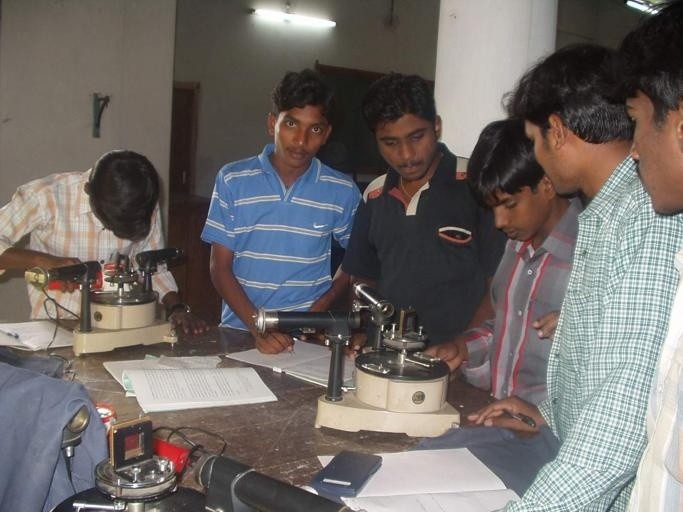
[100,352,279,415]
[316,447,522,512]
[223,333,357,390]
[0,319,74,351]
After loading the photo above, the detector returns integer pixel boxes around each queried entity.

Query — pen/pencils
[485,399,534,428]
[287,346,295,357]
[0,327,19,339]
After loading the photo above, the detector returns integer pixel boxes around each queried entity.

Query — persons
[0,144,210,337]
[595,0,683,512]
[466,39,683,512]
[298,69,508,382]
[198,67,364,358]
[419,116,587,405]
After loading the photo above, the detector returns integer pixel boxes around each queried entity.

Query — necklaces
[398,175,412,200]
